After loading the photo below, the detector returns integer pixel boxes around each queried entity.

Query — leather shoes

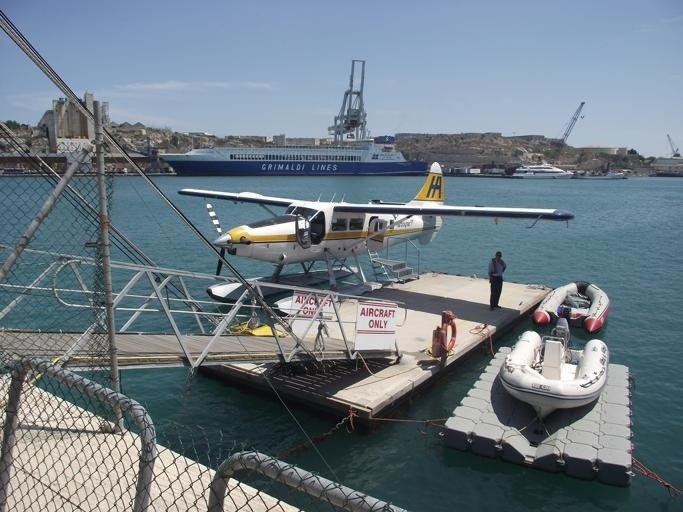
[490,305,501,310]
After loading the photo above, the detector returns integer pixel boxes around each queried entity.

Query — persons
[489,251,506,310]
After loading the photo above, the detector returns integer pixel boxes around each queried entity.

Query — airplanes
[178,163,575,316]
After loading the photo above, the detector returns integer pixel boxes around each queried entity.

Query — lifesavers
[439,320,456,352]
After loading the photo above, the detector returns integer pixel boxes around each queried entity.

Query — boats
[513,166,574,179]
[576,173,625,179]
[533,281,611,332]
[649,169,682,177]
[499,330,609,421]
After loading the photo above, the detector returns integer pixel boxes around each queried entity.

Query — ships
[160,139,428,176]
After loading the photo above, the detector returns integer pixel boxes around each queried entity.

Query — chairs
[535,336,566,376]
